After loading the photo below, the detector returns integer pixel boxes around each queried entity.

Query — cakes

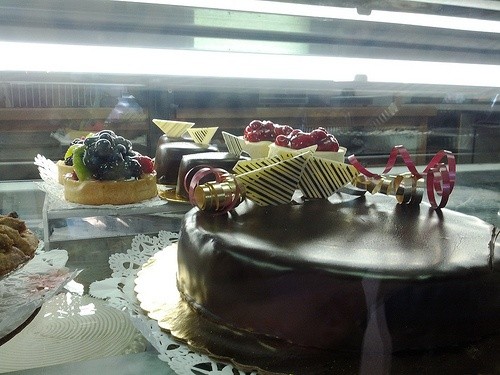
[237,120,294,158]
[152,118,196,148]
[266,125,348,164]
[63,129,159,204]
[179,144,500,375]
[153,126,219,185]
[58,136,86,186]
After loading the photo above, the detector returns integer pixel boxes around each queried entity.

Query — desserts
[0,210,40,277]
